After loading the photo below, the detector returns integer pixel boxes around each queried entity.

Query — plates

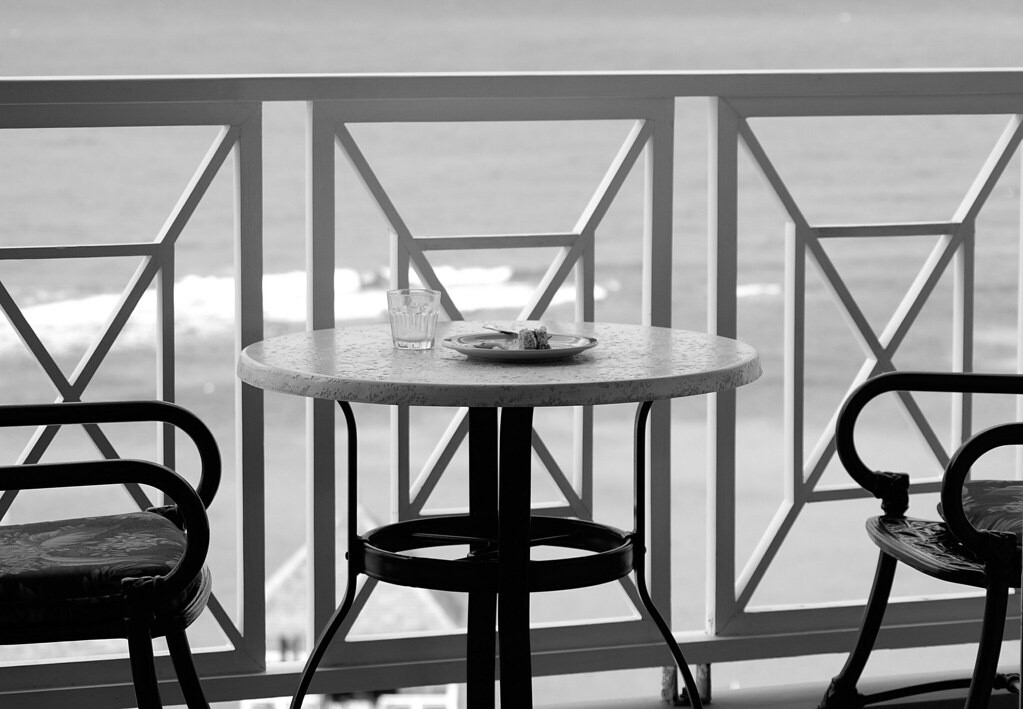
[441,333,599,360]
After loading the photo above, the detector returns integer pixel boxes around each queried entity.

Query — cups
[387,289,440,351]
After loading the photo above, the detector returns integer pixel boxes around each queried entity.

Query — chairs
[0,399,223,709]
[816,371,1023,709]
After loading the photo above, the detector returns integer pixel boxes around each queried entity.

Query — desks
[235,320,762,709]
